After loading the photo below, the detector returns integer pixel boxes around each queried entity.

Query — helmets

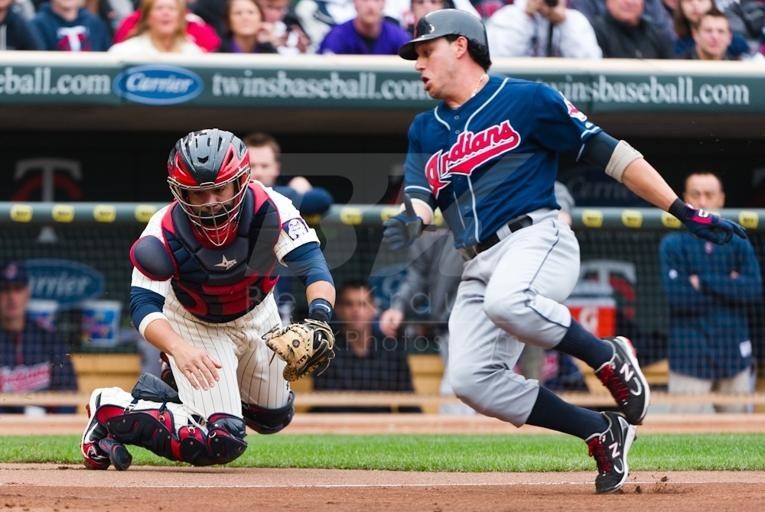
[167,127,252,246]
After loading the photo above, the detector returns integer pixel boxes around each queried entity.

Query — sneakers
[79,387,113,470]
[593,334,650,427]
[583,409,637,495]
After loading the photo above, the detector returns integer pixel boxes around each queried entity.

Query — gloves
[667,197,747,247]
[382,192,438,256]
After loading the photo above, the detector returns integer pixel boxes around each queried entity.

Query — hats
[1,257,29,286]
[398,9,493,69]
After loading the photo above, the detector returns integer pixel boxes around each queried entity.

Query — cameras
[543,0,558,7]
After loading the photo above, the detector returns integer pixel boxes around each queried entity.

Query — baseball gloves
[266,318,335,381]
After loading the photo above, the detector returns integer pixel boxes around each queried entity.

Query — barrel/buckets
[28,297,59,333]
[78,300,123,350]
